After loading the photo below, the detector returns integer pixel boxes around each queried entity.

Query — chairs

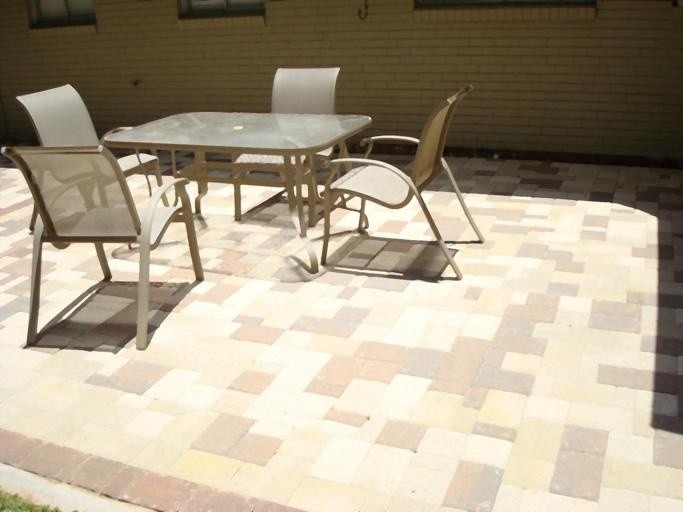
[1,145,204,350]
[316,84,485,280]
[231,66,357,238]
[15,84,175,235]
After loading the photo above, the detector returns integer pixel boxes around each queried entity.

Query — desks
[103,110,373,276]
